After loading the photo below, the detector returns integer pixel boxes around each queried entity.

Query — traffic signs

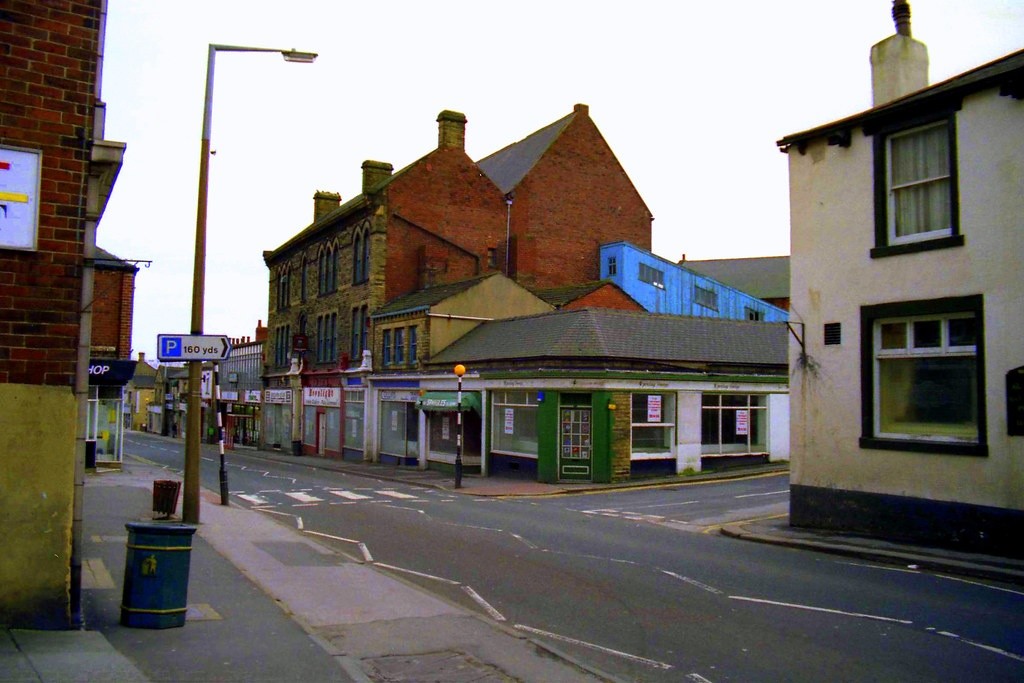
[157,334,234,361]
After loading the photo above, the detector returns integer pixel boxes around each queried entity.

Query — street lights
[182,43,320,524]
[454,364,466,489]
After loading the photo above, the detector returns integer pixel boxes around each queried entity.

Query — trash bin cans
[120,522,196,630]
